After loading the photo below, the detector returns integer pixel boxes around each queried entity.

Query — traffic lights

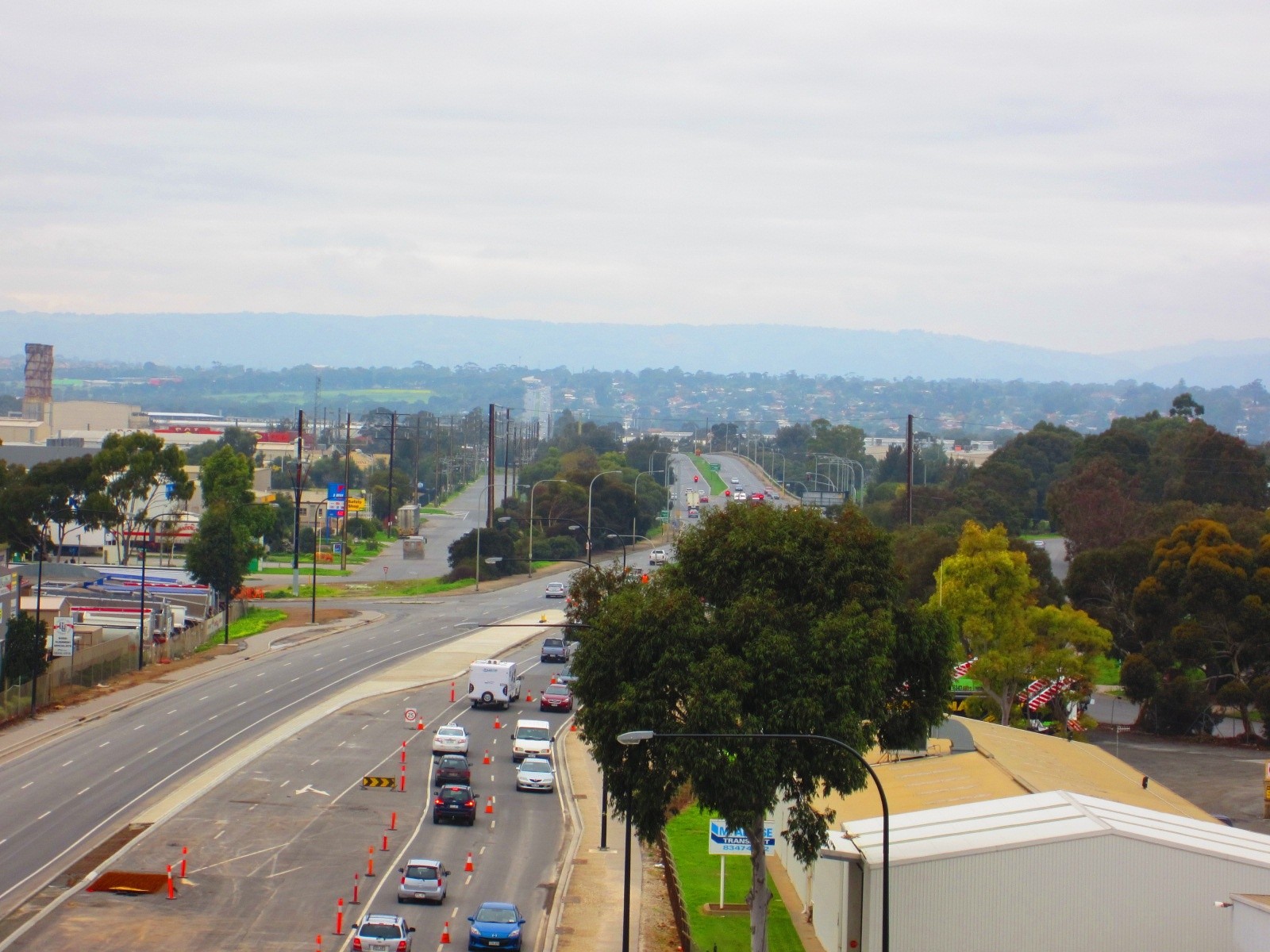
[759,493,764,500]
[694,476,698,483]
[725,490,731,496]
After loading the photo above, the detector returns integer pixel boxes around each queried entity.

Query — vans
[468,659,524,711]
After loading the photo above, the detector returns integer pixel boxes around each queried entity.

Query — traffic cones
[482,749,491,765]
[569,719,577,732]
[525,689,533,702]
[485,795,494,813]
[493,716,501,729]
[416,716,425,730]
[463,851,474,872]
[439,920,452,944]
[549,672,558,685]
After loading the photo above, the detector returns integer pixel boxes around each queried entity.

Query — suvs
[433,783,481,827]
[433,754,473,788]
[511,719,555,762]
[397,859,452,905]
[351,913,416,952]
[649,549,669,566]
[541,637,571,663]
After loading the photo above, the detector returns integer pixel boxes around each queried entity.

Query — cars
[735,484,744,491]
[467,902,527,952]
[730,477,740,483]
[432,722,472,757]
[685,488,709,518]
[546,582,568,598]
[515,758,557,793]
[670,493,679,501]
[734,493,764,504]
[625,567,643,582]
[540,683,573,714]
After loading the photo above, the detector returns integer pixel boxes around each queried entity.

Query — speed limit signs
[406,710,416,721]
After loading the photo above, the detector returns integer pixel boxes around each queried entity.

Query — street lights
[453,621,620,848]
[312,495,362,627]
[632,470,664,551]
[759,446,774,478]
[529,479,567,577]
[769,452,785,493]
[607,534,658,577]
[138,512,205,674]
[567,525,626,585]
[804,451,864,508]
[225,503,280,643]
[778,479,807,492]
[497,516,592,572]
[476,485,531,592]
[29,509,136,719]
[649,452,671,474]
[587,470,623,565]
[793,452,817,490]
[617,730,890,952]
